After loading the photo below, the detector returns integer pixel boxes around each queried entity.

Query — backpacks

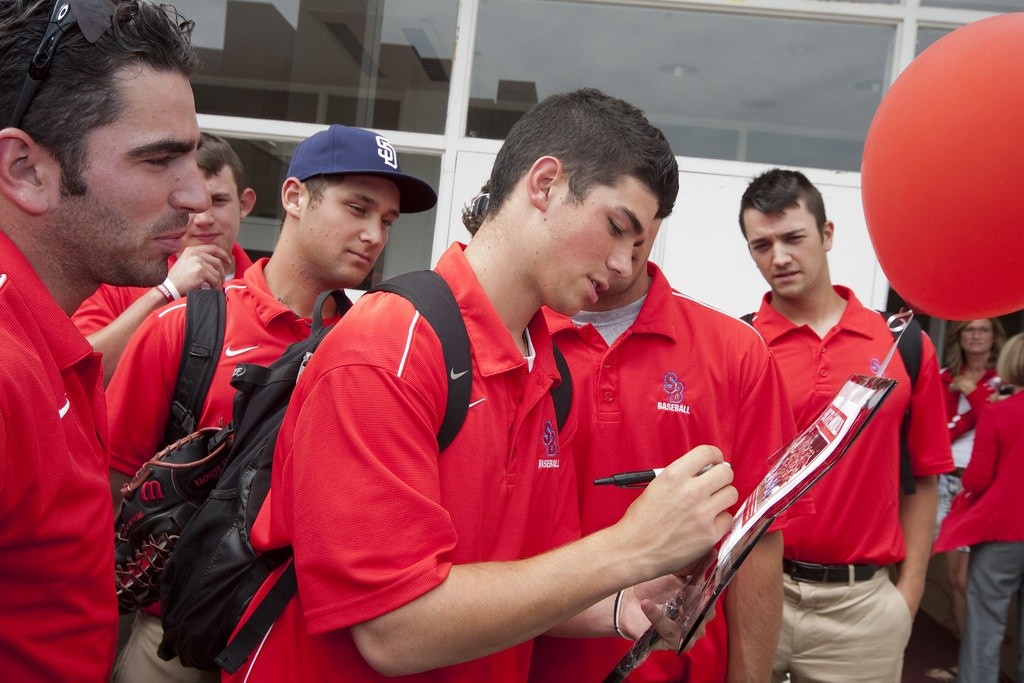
[159,270,572,671]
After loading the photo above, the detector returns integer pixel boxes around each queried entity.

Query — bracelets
[161,278,182,301]
[155,284,175,303]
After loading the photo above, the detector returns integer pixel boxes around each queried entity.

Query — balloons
[860,11,1024,323]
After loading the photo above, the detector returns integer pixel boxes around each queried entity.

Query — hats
[287,124,438,214]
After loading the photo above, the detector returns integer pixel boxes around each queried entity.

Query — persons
[924,316,1012,683]
[211,79,742,683]
[734,166,960,683]
[106,126,441,547]
[2,0,213,681]
[69,126,258,388]
[956,334,1024,682]
[539,132,797,681]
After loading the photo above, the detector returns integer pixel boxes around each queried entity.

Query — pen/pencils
[593,462,733,488]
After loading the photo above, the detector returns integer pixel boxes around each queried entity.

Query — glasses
[11,1,119,130]
